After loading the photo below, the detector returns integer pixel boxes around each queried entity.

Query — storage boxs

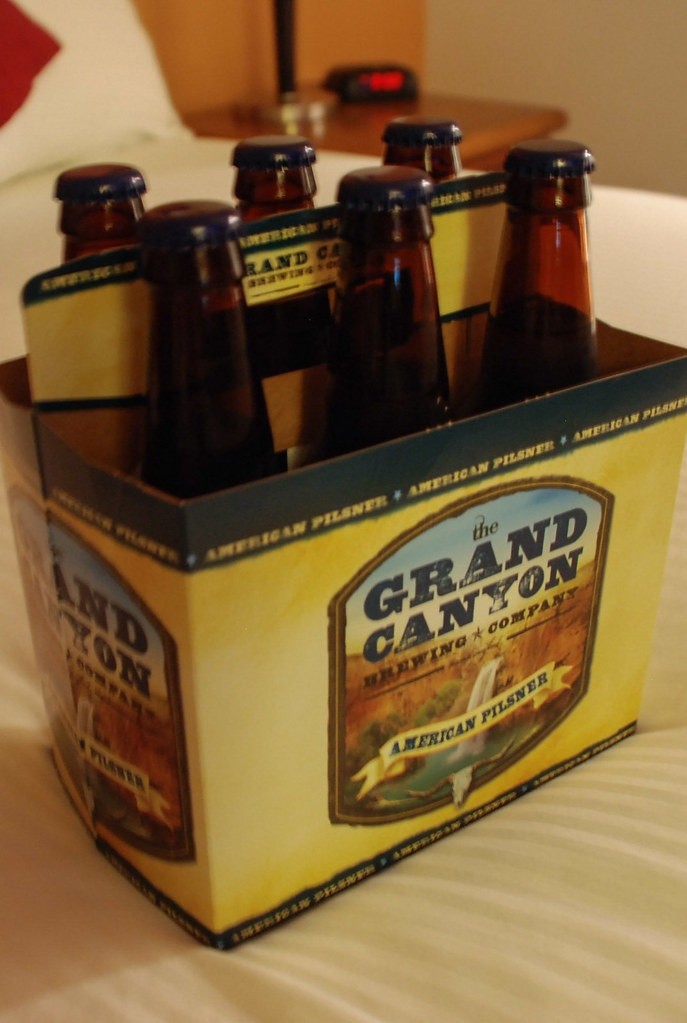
[0,318,687,952]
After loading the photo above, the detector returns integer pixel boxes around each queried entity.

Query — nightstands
[192,89,569,171]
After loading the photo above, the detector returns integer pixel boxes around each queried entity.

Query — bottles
[136,198,277,497]
[469,137,596,404]
[381,116,463,186]
[306,165,452,457]
[54,163,147,263]
[229,135,319,225]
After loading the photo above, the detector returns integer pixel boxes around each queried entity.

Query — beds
[0,0,687,1023]
